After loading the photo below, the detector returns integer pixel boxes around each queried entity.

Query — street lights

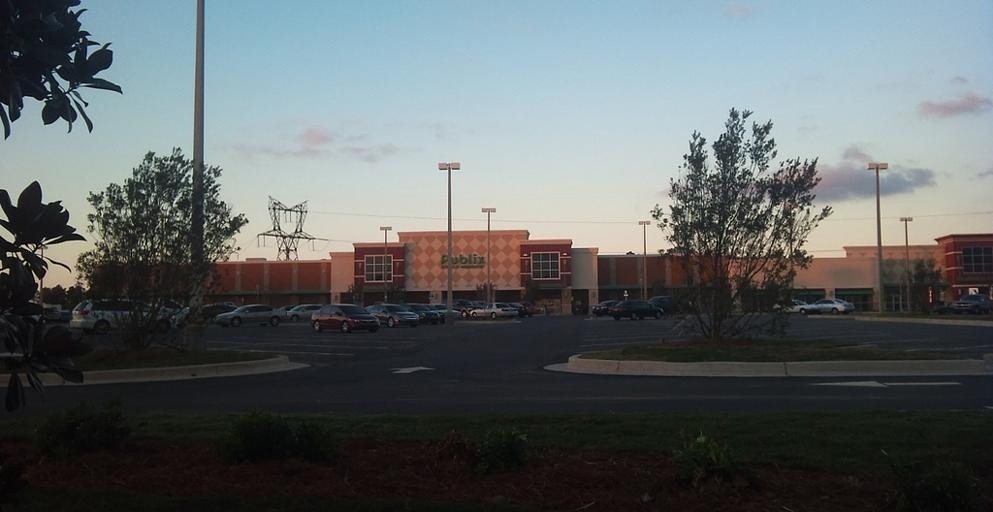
[866,162,888,316]
[379,227,392,304]
[35,246,47,307]
[638,220,650,300]
[481,208,495,304]
[899,217,912,312]
[438,162,460,327]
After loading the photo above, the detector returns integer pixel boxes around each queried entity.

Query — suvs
[69,299,157,335]
[951,294,990,315]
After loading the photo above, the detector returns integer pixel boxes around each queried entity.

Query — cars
[797,298,856,314]
[30,304,71,326]
[197,303,240,326]
[286,304,325,323]
[591,300,618,317]
[407,303,441,326]
[365,304,420,328]
[772,299,808,314]
[213,304,288,328]
[927,300,958,314]
[310,304,380,335]
[432,303,461,324]
[607,300,664,320]
[648,297,680,318]
[148,297,184,317]
[453,299,530,320]
